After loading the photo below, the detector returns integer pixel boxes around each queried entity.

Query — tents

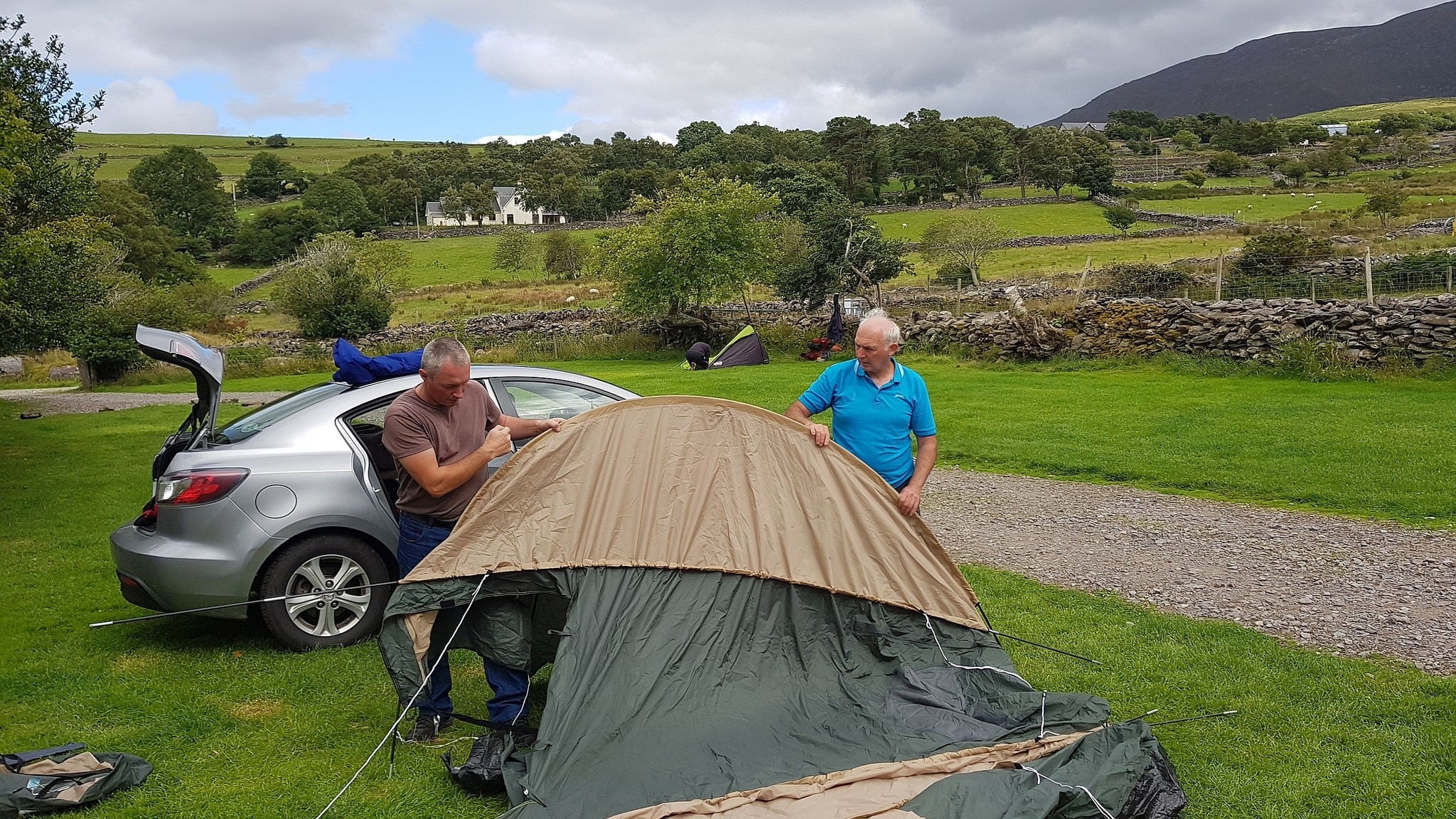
[680,325,769,371]
[375,400,1185,819]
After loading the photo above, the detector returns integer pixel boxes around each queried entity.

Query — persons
[381,337,568,746]
[685,342,712,371]
[785,306,937,516]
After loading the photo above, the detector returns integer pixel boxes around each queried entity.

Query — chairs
[351,424,401,506]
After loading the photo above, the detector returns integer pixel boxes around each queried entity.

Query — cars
[108,324,643,652]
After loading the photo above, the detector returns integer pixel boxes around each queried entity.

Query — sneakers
[405,710,453,741]
[689,362,696,371]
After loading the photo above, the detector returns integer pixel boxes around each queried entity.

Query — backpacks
[0,754,153,819]
